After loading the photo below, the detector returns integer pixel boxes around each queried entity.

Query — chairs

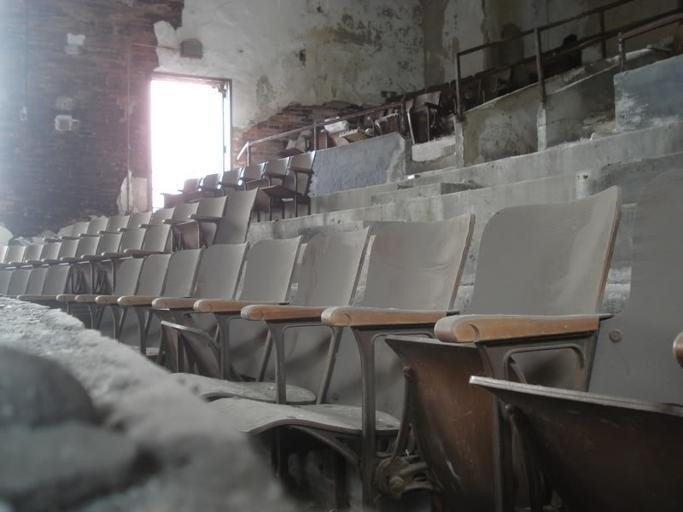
[176,235,303,382]
[283,44,568,163]
[152,240,249,378]
[117,248,203,373]
[0,270,12,297]
[31,266,71,309]
[95,254,171,355]
[197,226,375,400]
[75,258,144,339]
[7,269,32,299]
[2,186,260,266]
[434,169,683,512]
[16,268,48,303]
[159,152,316,221]
[208,212,475,512]
[321,184,623,511]
